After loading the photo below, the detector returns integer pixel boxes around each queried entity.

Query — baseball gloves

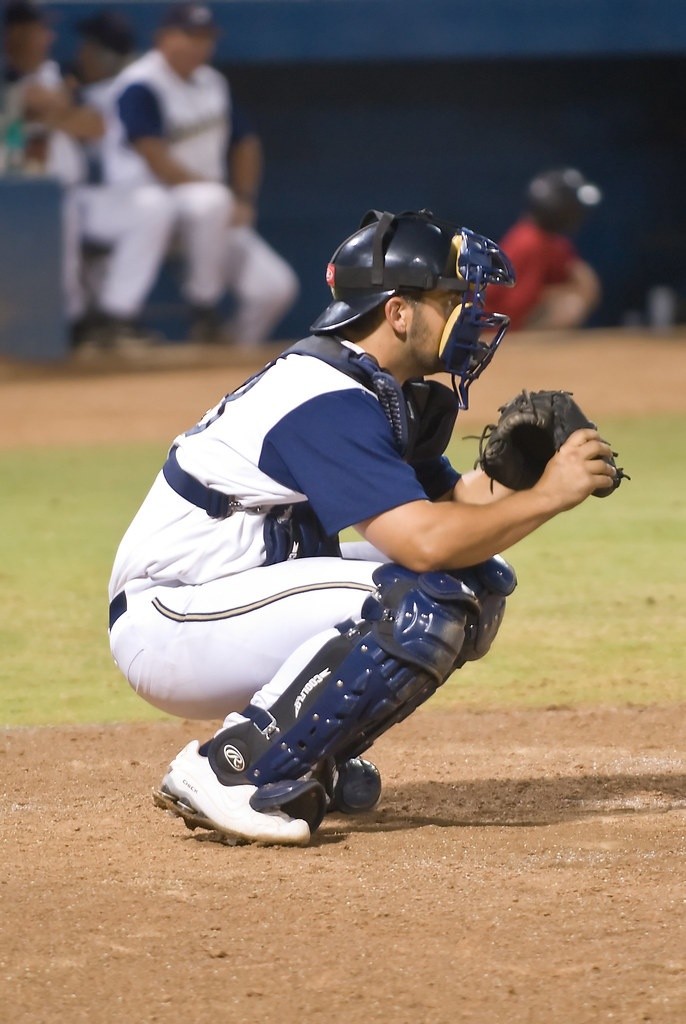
[476,390,630,499]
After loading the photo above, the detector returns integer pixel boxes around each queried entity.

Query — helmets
[528,169,589,228]
[310,209,470,333]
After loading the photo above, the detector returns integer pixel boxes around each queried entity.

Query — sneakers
[294,750,340,813]
[150,740,311,847]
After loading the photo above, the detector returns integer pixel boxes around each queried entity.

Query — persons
[483,165,607,335]
[108,214,618,848]
[0,0,295,363]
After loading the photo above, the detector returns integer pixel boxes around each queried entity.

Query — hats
[160,0,217,39]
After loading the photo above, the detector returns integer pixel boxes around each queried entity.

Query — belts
[109,591,127,631]
